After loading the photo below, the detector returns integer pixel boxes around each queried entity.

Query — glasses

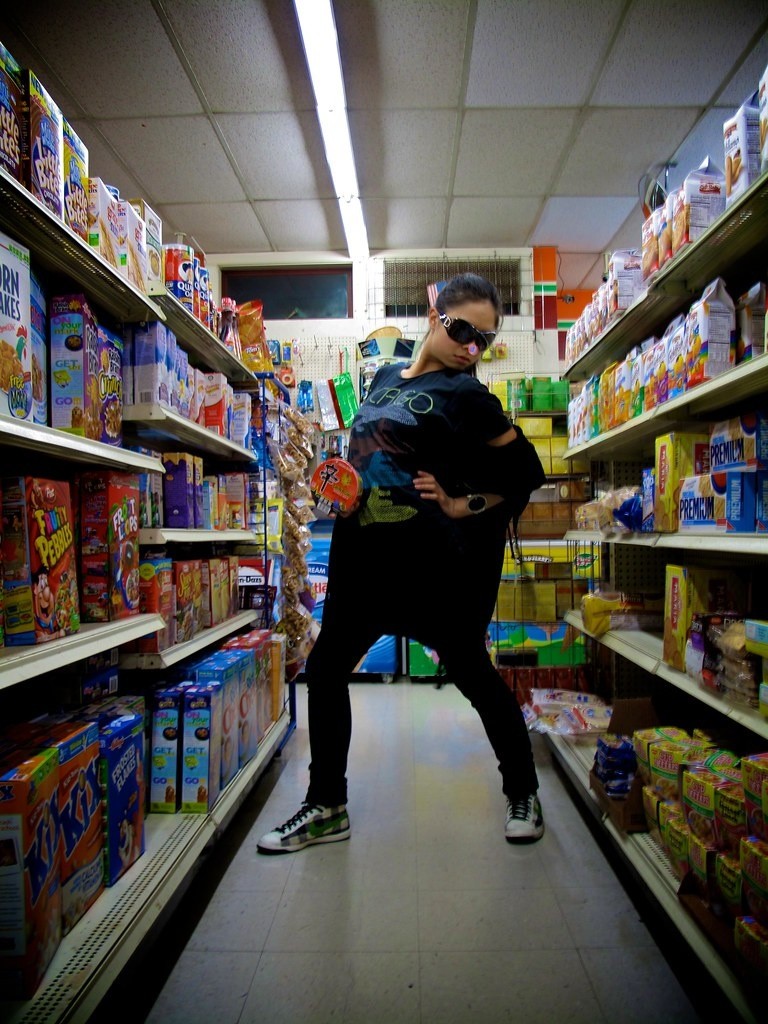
[436,307,497,352]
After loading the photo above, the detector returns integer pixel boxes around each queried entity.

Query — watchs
[467,495,487,514]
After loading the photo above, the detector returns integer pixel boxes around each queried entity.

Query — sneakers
[505,789,545,840]
[257,800,352,852]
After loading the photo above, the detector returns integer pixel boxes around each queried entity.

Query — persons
[257,272,549,855]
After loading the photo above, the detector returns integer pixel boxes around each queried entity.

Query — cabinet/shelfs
[545,172,768,1024]
[0,40,332,1024]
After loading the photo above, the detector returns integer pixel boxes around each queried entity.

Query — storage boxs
[615,417,767,536]
[488,367,592,539]
[661,563,768,721]
[578,697,768,1022]
[0,53,288,999]
